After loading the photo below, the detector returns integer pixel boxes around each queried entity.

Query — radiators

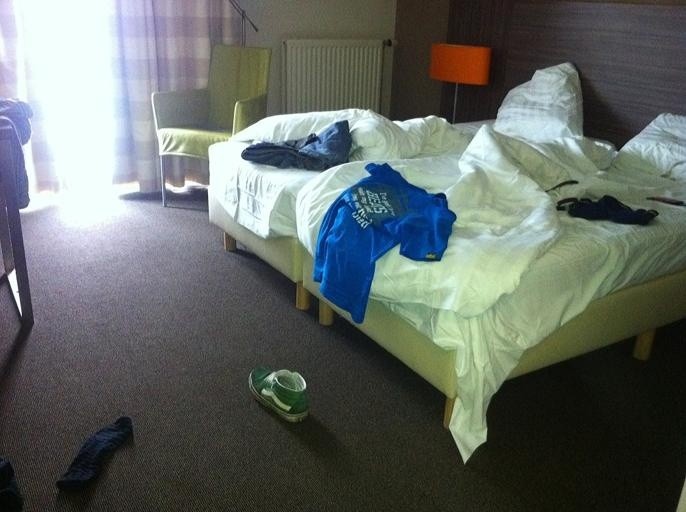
[279,37,392,115]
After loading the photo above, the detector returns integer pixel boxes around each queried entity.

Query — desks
[0,126,34,325]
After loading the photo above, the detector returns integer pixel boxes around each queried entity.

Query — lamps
[428,43,492,124]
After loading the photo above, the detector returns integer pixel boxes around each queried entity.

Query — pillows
[611,111,686,182]
[492,62,585,142]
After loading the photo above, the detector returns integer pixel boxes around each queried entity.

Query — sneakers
[249,366,311,428]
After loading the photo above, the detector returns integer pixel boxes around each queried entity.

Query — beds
[293,153,686,433]
[207,118,622,312]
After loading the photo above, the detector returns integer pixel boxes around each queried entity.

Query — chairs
[151,43,272,208]
